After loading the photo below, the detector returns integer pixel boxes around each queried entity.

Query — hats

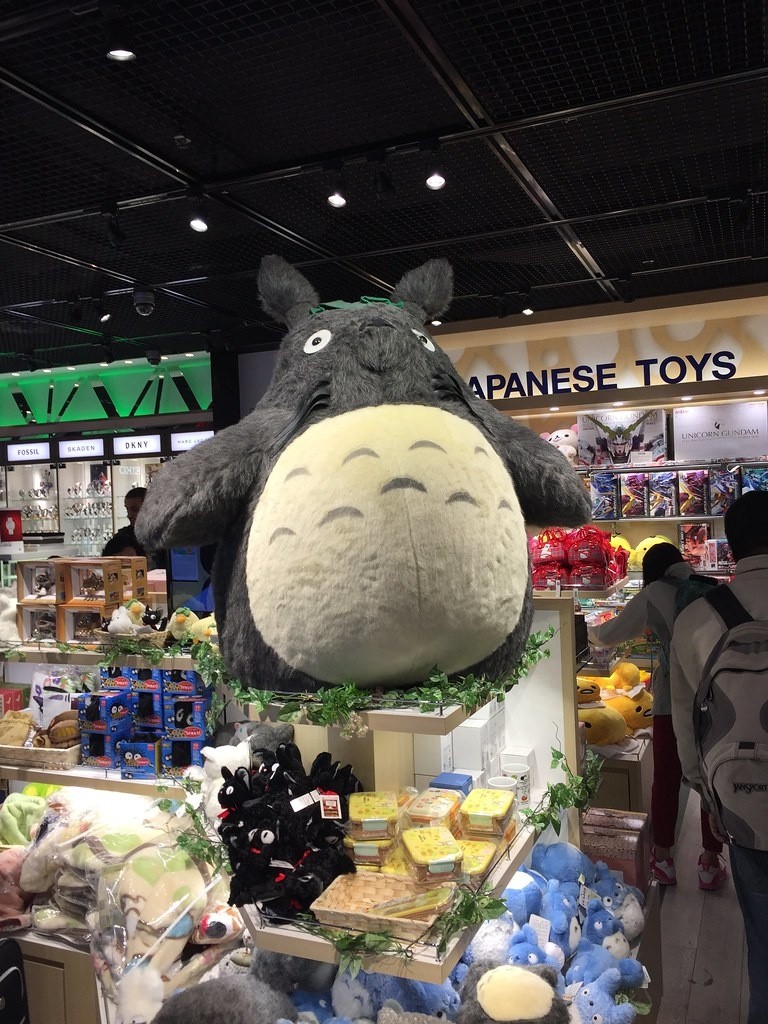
[642,542,685,588]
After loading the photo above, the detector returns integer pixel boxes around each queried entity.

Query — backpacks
[661,575,721,622]
[701,583,768,852]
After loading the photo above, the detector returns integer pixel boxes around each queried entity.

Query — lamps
[425,159,446,190]
[107,220,126,245]
[522,296,534,317]
[375,161,397,202]
[95,304,111,322]
[103,15,137,63]
[327,178,347,209]
[189,203,209,232]
[623,286,635,303]
[201,332,216,351]
[102,349,115,364]
[23,358,36,373]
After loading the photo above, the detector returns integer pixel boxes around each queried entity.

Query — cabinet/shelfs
[0,452,199,631]
[545,459,768,671]
[0,594,580,1023]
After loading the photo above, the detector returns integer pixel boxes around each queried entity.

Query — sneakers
[697,852,728,890]
[649,845,677,885]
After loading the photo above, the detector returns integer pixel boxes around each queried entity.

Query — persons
[589,544,729,891]
[669,489,768,1024]
[102,487,152,558]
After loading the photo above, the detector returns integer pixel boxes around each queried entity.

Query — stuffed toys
[0,565,105,648]
[131,255,592,696]
[107,599,222,657]
[0,664,657,1024]
[522,421,688,601]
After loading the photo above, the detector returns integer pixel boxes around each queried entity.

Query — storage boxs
[15,554,215,779]
[585,467,767,568]
[0,683,31,719]
[309,692,537,945]
[582,806,648,892]
